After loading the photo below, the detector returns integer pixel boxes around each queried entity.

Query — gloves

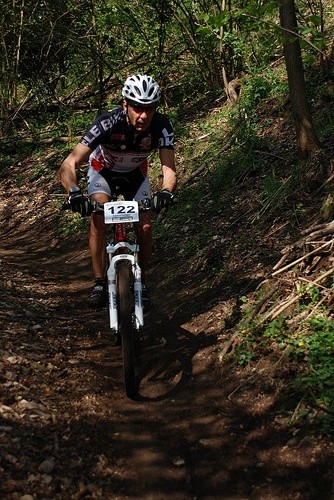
[151,189,174,211]
[68,191,93,217]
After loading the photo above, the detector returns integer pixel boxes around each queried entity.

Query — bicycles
[60,196,178,400]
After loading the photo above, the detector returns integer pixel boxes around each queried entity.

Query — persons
[59,75,177,303]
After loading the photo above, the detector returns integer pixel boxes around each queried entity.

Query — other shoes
[90,277,106,305]
[140,285,151,313]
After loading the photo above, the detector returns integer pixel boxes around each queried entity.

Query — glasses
[126,99,156,109]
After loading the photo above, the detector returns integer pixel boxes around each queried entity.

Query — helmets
[121,75,162,105]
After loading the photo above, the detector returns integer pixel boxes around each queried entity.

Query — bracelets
[161,188,170,192]
